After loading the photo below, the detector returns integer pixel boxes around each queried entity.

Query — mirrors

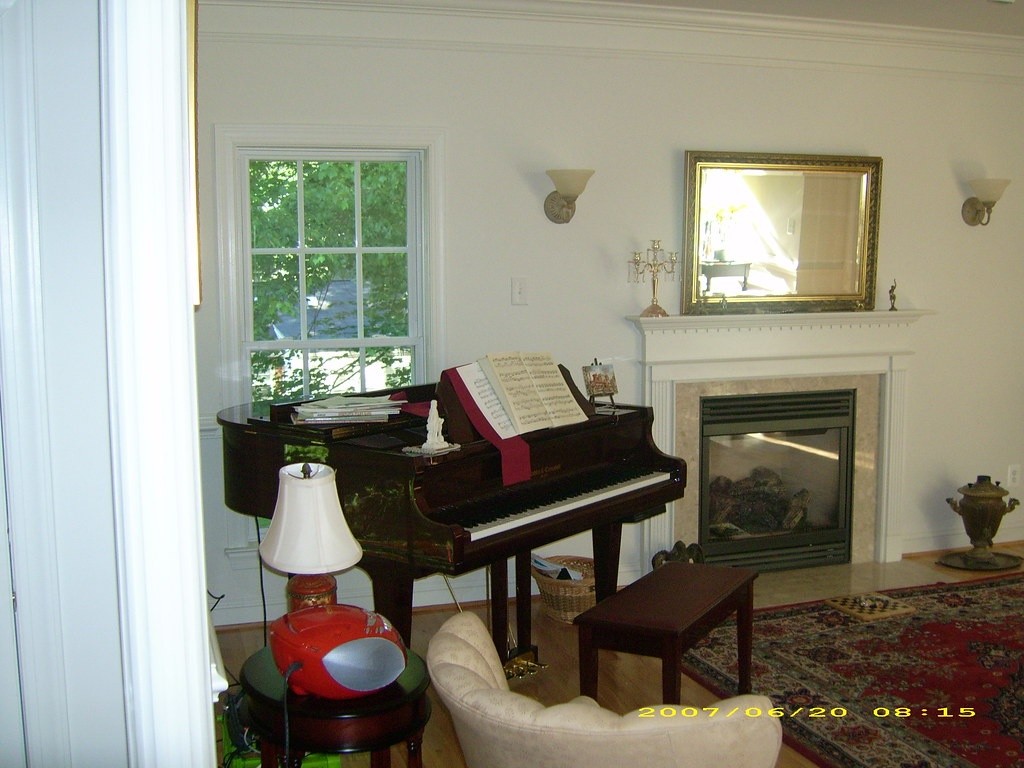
[683,150,882,317]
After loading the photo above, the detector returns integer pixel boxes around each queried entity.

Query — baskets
[531,556,597,625]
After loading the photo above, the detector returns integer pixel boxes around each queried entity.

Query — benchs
[572,561,760,710]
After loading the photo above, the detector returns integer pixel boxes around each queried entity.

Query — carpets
[682,571,1024,768]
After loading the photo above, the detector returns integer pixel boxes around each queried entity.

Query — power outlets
[1007,464,1020,486]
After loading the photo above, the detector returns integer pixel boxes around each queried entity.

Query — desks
[241,645,432,768]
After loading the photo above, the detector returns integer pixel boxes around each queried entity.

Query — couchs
[427,611,782,768]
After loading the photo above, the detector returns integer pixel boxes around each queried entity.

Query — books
[478,352,589,436]
[530,555,583,581]
[290,395,406,424]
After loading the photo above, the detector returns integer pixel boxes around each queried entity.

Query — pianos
[217,359,688,700]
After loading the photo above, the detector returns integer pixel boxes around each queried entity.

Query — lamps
[962,178,1012,227]
[543,167,595,224]
[259,462,363,611]
